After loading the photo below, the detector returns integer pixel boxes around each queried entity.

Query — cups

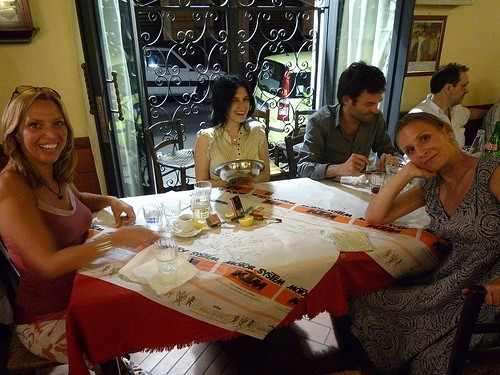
[153,237,178,276]
[164,199,182,227]
[195,180,212,203]
[191,196,212,221]
[178,213,194,232]
[370,155,422,197]
[142,202,163,229]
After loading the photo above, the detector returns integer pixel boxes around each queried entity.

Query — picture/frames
[407,15,449,76]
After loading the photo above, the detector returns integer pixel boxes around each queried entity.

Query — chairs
[145,109,315,192]
[1,254,63,374]
[446,284,500,374]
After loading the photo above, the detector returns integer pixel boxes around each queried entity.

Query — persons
[0,86,152,375]
[408,62,500,152]
[348,112,500,375]
[194,74,271,188]
[297,61,403,180]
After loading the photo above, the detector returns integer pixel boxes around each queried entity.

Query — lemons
[226,211,235,219]
[238,215,253,226]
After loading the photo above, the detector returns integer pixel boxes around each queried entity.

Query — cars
[144,45,234,101]
[251,51,317,150]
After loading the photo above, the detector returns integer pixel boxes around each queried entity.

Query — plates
[170,226,202,237]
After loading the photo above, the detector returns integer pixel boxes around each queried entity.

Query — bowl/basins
[211,158,266,187]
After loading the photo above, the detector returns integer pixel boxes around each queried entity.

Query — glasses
[5,85,62,110]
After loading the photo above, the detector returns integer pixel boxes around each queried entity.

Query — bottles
[485,121,500,153]
[469,129,486,153]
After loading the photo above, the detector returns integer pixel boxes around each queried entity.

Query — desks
[65,176,445,375]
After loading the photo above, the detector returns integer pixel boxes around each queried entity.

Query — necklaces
[231,136,237,140]
[42,179,65,200]
